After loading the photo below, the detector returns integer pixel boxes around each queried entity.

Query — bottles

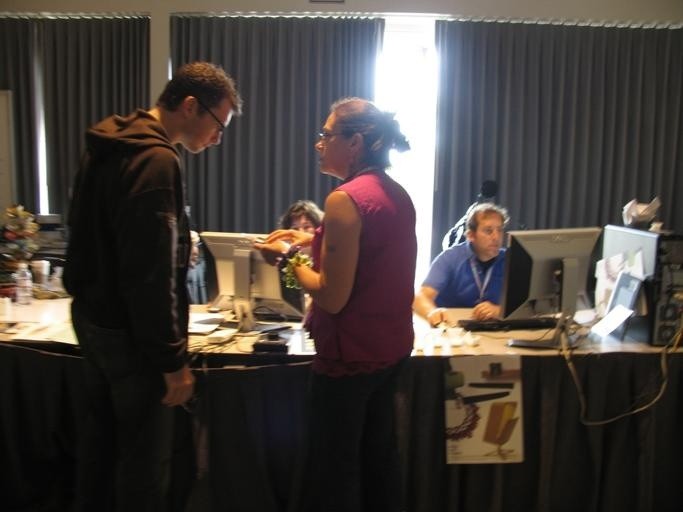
[16,264,33,306]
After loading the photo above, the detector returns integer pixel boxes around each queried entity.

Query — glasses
[315,128,346,140]
[198,97,227,131]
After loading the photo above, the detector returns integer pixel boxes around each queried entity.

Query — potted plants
[0,203,43,304]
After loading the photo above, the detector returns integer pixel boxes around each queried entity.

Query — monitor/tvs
[200,231,306,334]
[501,227,602,350]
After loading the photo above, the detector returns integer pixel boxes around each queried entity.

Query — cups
[33,260,51,284]
[1,297,13,322]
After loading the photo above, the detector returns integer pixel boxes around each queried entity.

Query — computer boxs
[603,224,683,347]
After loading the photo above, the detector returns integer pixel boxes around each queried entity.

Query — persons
[411,201,507,329]
[70,60,246,511]
[249,95,420,512]
[279,198,326,234]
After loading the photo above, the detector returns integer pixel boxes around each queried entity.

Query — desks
[0,293,682,512]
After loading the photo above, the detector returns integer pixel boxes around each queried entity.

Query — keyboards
[231,313,303,321]
[458,317,557,331]
